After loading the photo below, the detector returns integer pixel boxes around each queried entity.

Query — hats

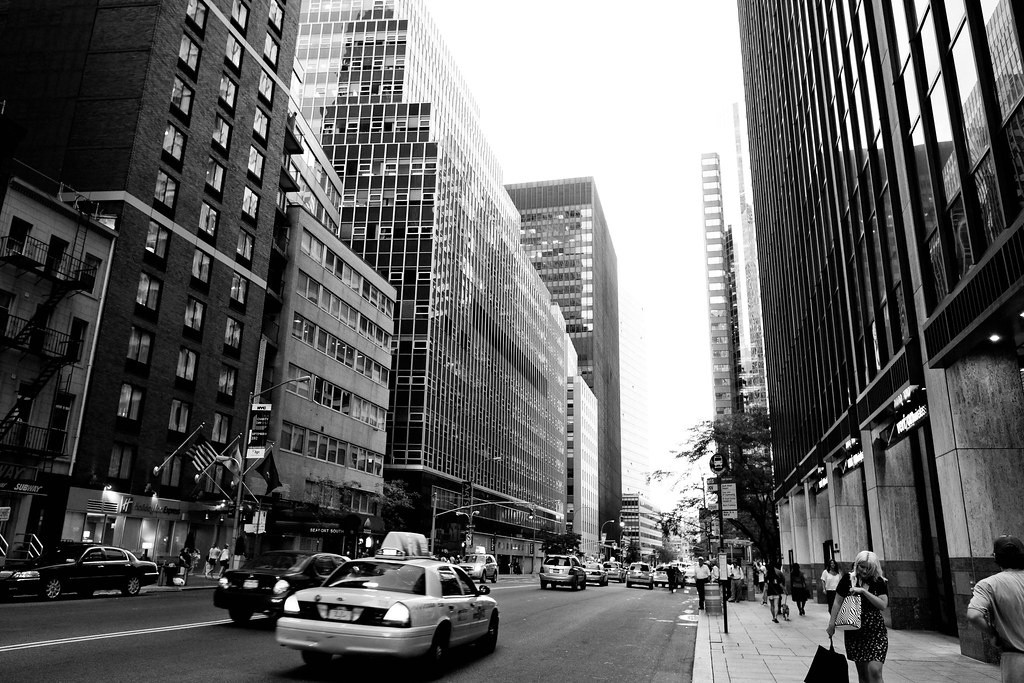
[995,534,1024,556]
[698,557,704,560]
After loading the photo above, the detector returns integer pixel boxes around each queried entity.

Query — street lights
[228,376,312,571]
[532,499,562,573]
[468,456,502,554]
[601,520,614,564]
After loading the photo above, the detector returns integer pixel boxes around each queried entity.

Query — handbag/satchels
[835,572,861,630]
[804,635,849,683]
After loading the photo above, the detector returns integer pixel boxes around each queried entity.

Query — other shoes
[736,600,739,602]
[799,610,801,615]
[772,619,778,623]
[205,573,207,579]
[801,608,805,615]
[212,576,216,578]
[728,600,735,602]
[698,607,704,609]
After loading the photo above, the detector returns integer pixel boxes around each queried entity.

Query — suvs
[457,552,499,584]
[539,553,587,592]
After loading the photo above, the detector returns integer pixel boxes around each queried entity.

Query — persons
[966,534,1024,683]
[179,541,230,580]
[790,563,808,615]
[821,559,844,615]
[439,553,462,564]
[695,554,786,623]
[515,561,521,575]
[666,565,675,593]
[826,551,890,683]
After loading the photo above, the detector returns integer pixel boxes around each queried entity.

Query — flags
[220,441,243,480]
[182,430,218,471]
[254,449,283,496]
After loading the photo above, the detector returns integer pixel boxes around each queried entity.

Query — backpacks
[793,577,802,587]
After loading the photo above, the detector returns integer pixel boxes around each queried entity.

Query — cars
[581,557,696,590]
[275,529,500,681]
[213,549,359,623]
[0,543,160,602]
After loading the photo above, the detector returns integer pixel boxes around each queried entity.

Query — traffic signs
[465,524,476,529]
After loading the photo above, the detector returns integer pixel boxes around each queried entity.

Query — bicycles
[668,575,676,593]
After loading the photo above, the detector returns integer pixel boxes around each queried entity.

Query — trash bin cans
[703,583,722,612]
[164,566,178,587]
